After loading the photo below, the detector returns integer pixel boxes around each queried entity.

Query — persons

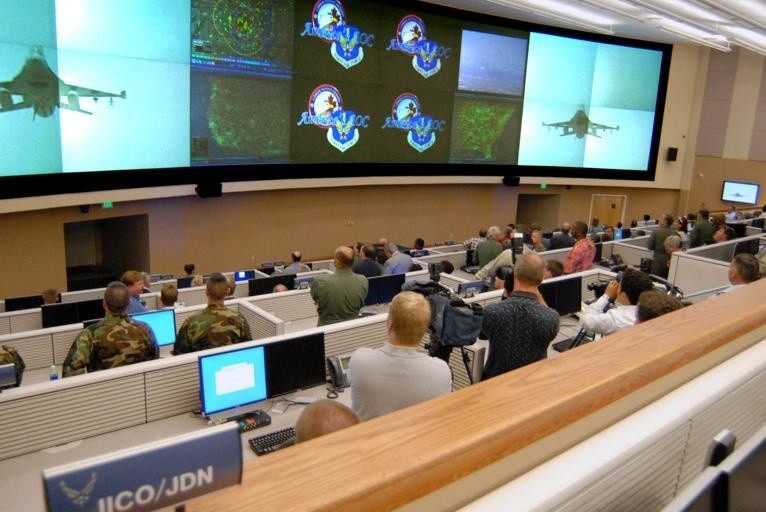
[296,397,363,446]
[40,289,58,304]
[167,273,257,354]
[347,289,452,421]
[64,280,171,375]
[259,237,460,343]
[0,345,25,388]
[461,206,765,330]
[480,254,553,384]
[118,268,187,315]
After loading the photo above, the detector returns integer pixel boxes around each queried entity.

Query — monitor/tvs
[198,332,326,424]
[538,276,581,316]
[364,273,405,306]
[721,181,760,205]
[593,244,602,262]
[128,309,177,347]
[177,263,312,296]
[5,294,45,312]
[733,239,759,258]
[41,299,106,328]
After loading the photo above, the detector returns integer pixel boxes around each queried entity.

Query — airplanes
[0,41,130,121]
[540,103,622,141]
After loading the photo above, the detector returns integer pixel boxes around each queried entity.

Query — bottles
[50,364,58,381]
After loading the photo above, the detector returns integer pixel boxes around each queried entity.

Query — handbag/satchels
[641,257,656,274]
[404,282,484,348]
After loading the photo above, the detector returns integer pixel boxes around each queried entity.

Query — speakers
[667,147,678,161]
[196,181,222,199]
[503,175,520,187]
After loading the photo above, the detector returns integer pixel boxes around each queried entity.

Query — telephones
[327,352,351,389]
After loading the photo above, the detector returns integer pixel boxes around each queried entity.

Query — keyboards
[553,334,592,352]
[249,426,296,455]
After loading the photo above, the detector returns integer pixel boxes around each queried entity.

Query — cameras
[588,280,615,304]
[496,233,524,292]
